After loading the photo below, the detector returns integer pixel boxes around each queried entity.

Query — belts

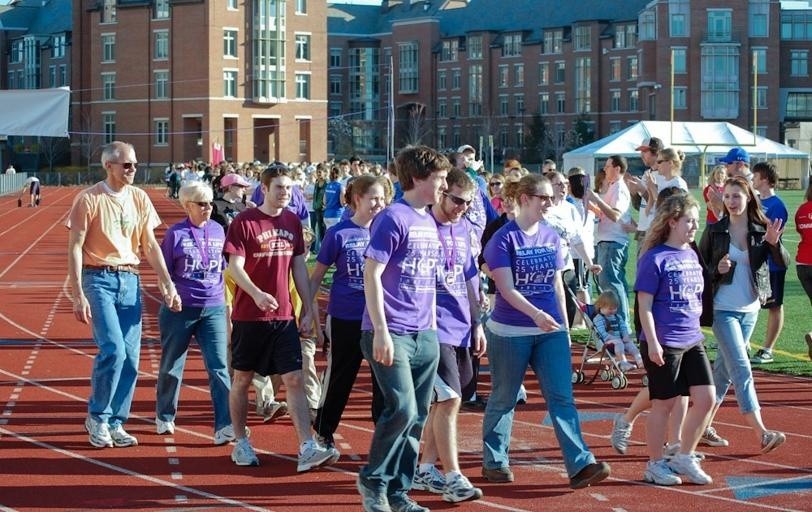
[86,265,139,274]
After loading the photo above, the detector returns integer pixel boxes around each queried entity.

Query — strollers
[562,265,651,391]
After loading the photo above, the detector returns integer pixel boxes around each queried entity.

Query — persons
[795,175,812,360]
[65,142,182,448]
[5,165,16,174]
[19,176,41,208]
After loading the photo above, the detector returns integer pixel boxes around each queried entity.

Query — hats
[636,137,663,152]
[458,145,475,153]
[220,174,250,188]
[719,148,749,163]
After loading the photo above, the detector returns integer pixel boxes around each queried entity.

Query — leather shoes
[570,462,610,489]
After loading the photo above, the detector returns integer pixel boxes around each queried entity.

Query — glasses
[122,163,137,168]
[192,201,214,206]
[531,195,555,200]
[491,182,501,185]
[657,159,669,165]
[443,191,471,205]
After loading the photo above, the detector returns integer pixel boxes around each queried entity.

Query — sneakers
[357,467,514,512]
[644,427,728,486]
[750,349,773,363]
[264,402,288,423]
[296,433,340,473]
[156,415,175,434]
[611,413,632,454]
[621,361,644,370]
[761,432,786,453]
[85,416,138,448]
[214,423,259,466]
